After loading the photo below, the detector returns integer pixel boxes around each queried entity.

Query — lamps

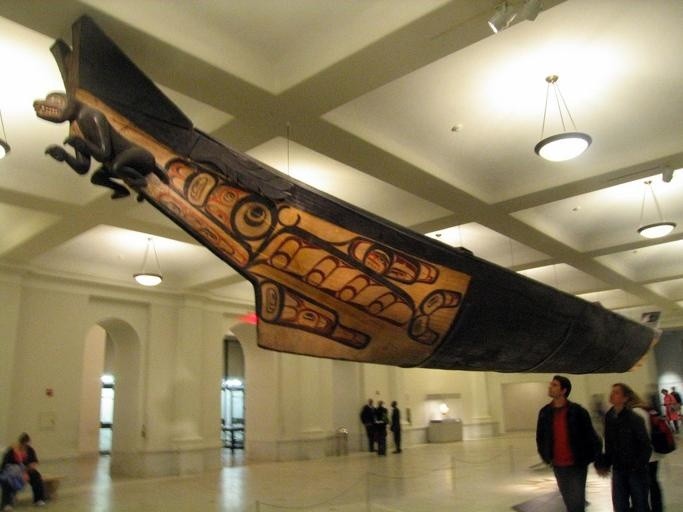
[534,75,592,162]
[522,0,542,21]
[637,181,676,239]
[0,110,10,158]
[488,0,516,34]
[133,237,163,285]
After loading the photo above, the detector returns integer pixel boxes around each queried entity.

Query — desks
[427,419,463,443]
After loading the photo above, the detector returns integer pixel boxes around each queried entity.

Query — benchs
[40,474,63,498]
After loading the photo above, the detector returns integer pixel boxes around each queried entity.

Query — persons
[661,383,682,433]
[630,388,676,512]
[535,375,602,512]
[603,383,655,512]
[0,432,47,511]
[360,397,402,457]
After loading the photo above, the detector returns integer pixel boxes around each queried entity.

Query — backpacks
[625,403,678,454]
